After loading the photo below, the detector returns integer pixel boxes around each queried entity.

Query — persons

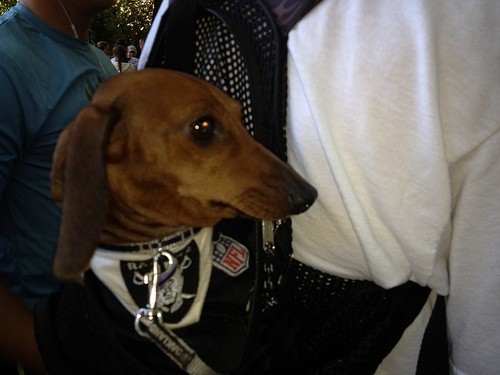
[0,0,500,375]
[110,45,134,74]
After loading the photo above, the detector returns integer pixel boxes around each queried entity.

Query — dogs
[48,64,322,374]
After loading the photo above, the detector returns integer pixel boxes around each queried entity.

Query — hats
[128,45,137,54]
